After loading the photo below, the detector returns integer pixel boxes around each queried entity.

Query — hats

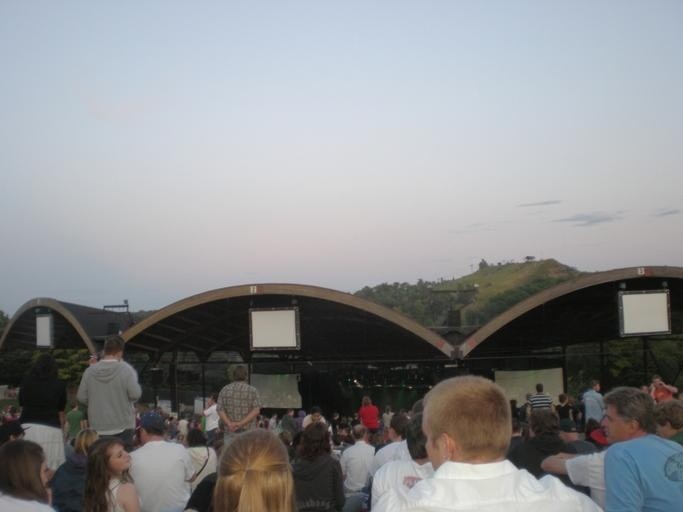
[136,414,162,429]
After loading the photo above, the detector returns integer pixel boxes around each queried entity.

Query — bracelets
[225,422,231,426]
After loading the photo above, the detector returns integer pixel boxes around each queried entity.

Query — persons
[215,365,263,446]
[75,336,143,454]
[17,353,68,471]
[1,373,681,512]
